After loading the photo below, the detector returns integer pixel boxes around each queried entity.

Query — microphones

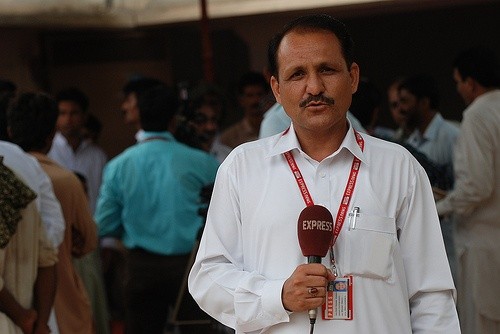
[298,205,333,325]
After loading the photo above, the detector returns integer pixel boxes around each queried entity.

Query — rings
[309,287,319,296]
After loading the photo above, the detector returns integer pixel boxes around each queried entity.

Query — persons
[395,71,462,282]
[187,13,463,334]
[119,68,416,215]
[435,51,500,334]
[0,140,66,334]
[5,90,99,334]
[45,90,111,333]
[91,77,222,334]
